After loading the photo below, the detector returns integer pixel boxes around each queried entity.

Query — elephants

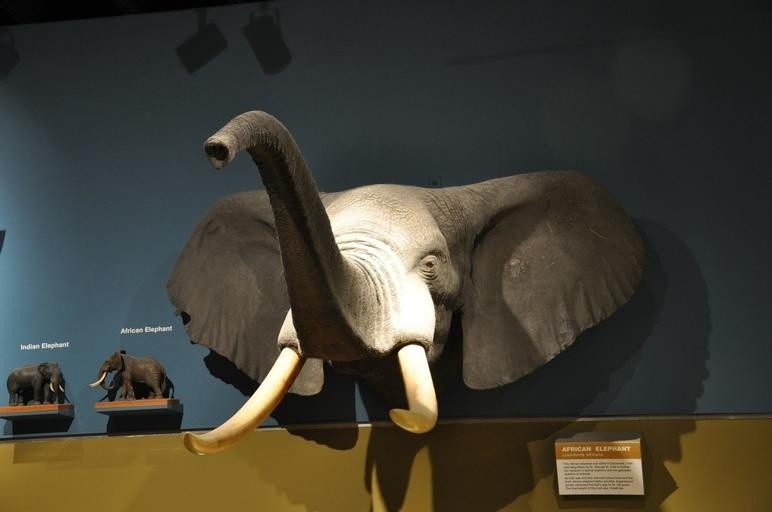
[89,347,166,401]
[7,362,65,406]
[162,110,648,455]
